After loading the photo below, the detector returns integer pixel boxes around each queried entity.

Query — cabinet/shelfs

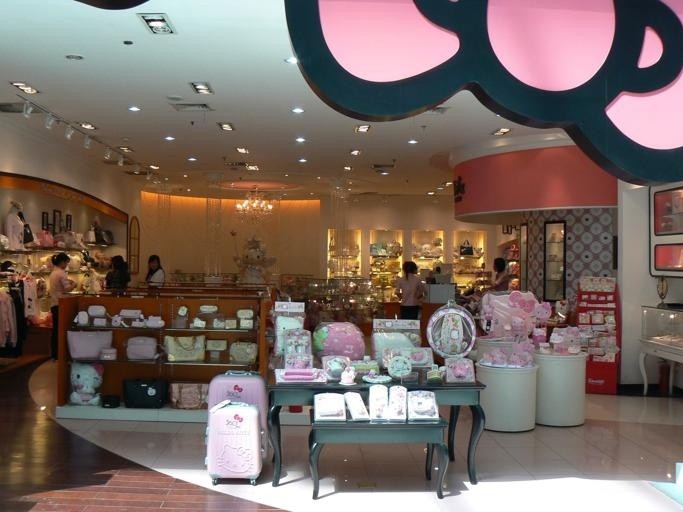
[543,220,566,302]
[369,254,401,274]
[330,254,363,278]
[54,294,268,423]
[574,279,619,397]
[453,254,483,275]
[519,223,528,293]
[637,303,682,396]
[412,253,444,276]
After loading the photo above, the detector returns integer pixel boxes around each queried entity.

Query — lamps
[16,94,160,182]
[233,185,280,226]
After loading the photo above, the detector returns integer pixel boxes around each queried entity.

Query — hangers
[2,264,32,298]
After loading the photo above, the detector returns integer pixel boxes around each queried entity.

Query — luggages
[205,371,269,460]
[205,401,263,485]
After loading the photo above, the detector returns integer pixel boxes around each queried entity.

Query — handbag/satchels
[67,305,165,361]
[19,213,33,243]
[86,226,96,242]
[169,382,207,409]
[165,304,258,365]
[123,379,166,408]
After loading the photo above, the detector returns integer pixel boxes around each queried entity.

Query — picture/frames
[66,213,71,231]
[40,211,49,230]
[52,209,62,237]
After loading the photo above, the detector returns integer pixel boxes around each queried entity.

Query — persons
[88,254,131,294]
[47,253,76,362]
[478,258,509,291]
[143,255,166,290]
[5,203,24,251]
[394,262,427,319]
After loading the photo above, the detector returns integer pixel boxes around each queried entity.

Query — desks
[306,407,452,500]
[263,367,487,490]
[467,334,587,434]
